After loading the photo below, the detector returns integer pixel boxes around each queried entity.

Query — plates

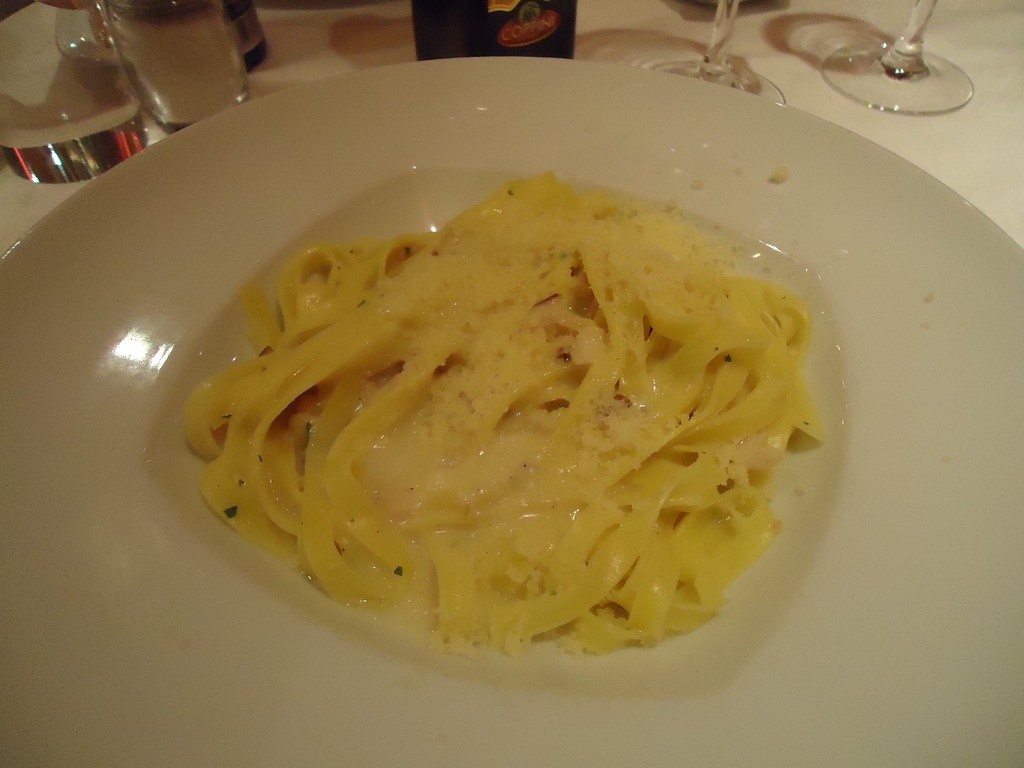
[0,55,1024,768]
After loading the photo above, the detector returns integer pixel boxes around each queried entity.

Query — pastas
[181,172,826,658]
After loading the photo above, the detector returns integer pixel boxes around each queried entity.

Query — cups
[106,0,248,136]
[0,0,148,181]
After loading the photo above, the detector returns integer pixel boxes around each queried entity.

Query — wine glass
[822,0,974,114]
[630,0,787,105]
